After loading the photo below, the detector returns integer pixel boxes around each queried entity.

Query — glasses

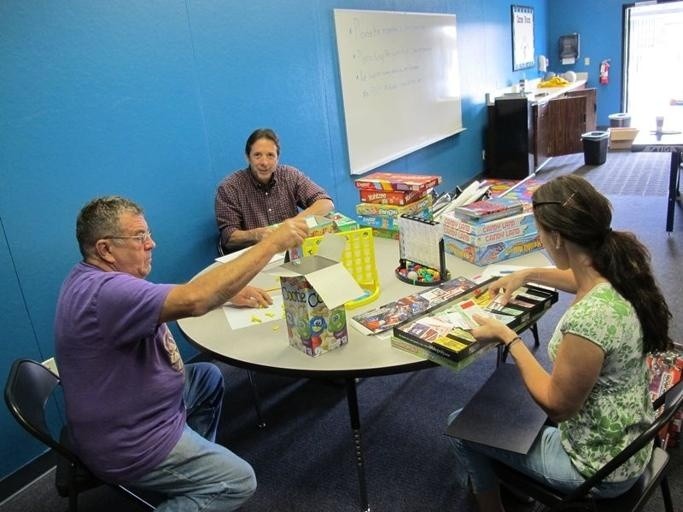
[103,231,151,242]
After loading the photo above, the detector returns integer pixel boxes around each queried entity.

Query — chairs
[488,382,683,512]
[5,357,159,512]
[216,235,292,428]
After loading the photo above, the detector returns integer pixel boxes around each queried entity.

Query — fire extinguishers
[600,58,611,85]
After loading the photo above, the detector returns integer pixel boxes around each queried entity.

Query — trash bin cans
[581,131,609,166]
[608,113,630,127]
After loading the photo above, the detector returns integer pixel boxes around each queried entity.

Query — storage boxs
[608,127,639,149]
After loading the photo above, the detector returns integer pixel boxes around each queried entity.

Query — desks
[631,112,683,232]
[177,236,556,512]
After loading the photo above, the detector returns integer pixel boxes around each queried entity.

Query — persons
[445,173,674,512]
[53,196,310,512]
[214,128,336,251]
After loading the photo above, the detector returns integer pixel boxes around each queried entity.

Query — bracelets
[506,336,522,353]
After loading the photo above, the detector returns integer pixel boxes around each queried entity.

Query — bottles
[519,79,526,97]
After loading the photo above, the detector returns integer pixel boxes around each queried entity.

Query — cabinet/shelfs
[487,84,597,180]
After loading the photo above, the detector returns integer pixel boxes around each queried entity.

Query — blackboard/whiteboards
[332,8,467,174]
